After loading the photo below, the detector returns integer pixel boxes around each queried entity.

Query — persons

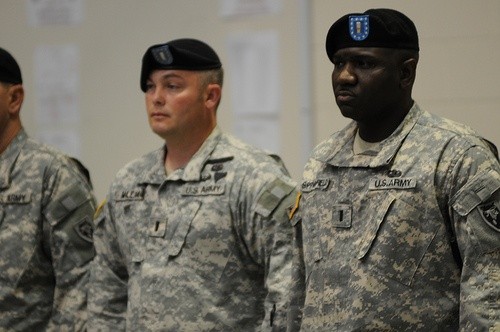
[0,46,97,332]
[86,37,299,332]
[287,8,500,332]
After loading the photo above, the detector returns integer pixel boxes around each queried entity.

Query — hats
[140,38,222,94]
[325,8,420,61]
